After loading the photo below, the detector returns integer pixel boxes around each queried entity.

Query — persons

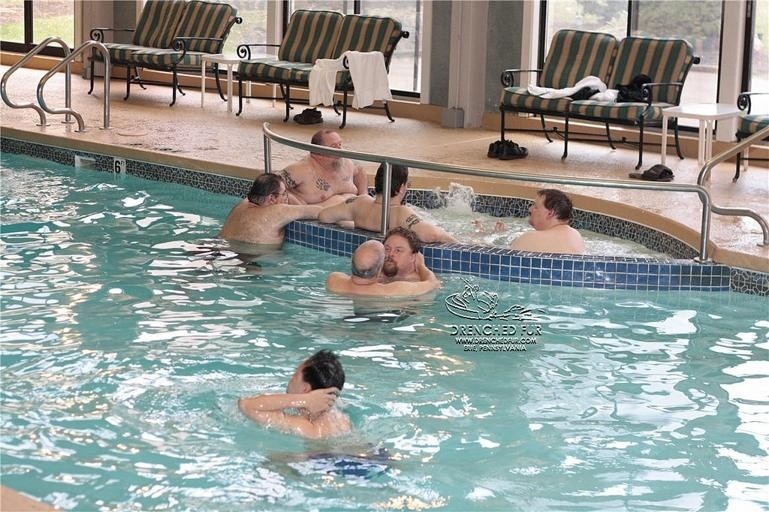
[326,240,439,307]
[510,189,587,255]
[377,225,422,283]
[318,163,507,244]
[277,130,368,209]
[238,348,353,440]
[219,173,324,255]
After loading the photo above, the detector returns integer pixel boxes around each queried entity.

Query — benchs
[500,31,699,170]
[737,91,769,182]
[88,0,241,107]
[236,10,408,129]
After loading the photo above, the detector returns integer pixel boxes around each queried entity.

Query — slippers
[629,164,674,181]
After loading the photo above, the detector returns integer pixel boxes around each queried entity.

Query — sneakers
[487,140,528,160]
[293,109,323,124]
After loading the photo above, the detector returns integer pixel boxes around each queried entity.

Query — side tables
[199,54,279,112]
[662,102,750,172]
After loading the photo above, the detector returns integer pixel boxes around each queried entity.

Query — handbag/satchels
[615,75,652,101]
